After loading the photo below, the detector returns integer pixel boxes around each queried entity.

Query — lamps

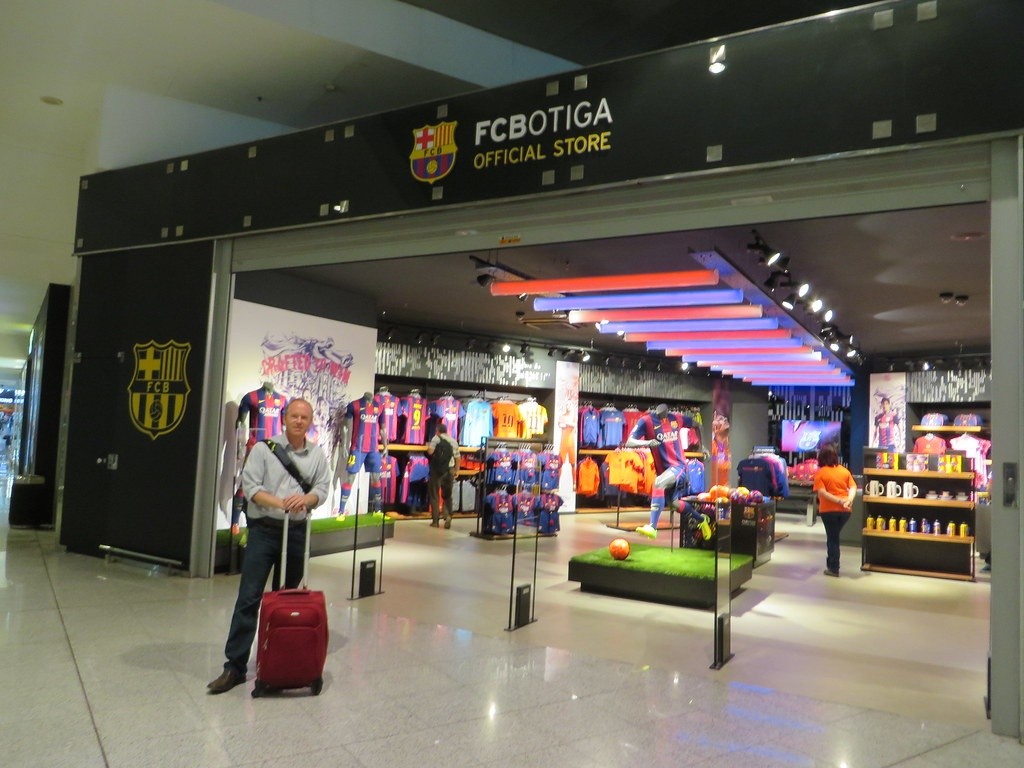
[746,229,870,369]
[516,295,529,302]
[383,331,724,378]
[888,357,989,372]
[937,292,969,306]
[477,273,496,288]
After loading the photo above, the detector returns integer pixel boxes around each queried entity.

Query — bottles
[960,523,969,538]
[889,516,896,531]
[899,517,907,532]
[867,515,874,529]
[947,521,956,537]
[876,515,885,530]
[909,518,916,532]
[933,519,940,535]
[921,517,930,533]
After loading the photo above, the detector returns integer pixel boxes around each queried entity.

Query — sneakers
[636,525,658,539]
[230,523,240,535]
[372,510,391,520]
[698,514,712,540]
[335,513,345,522]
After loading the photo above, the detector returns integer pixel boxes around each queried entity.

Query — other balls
[685,484,772,552]
[611,539,630,559]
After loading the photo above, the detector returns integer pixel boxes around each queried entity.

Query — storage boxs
[875,451,963,473]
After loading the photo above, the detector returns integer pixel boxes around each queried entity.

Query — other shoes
[824,569,840,578]
[429,519,439,528]
[444,517,452,529]
[979,564,991,574]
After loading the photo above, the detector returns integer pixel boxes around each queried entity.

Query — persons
[625,404,711,540]
[813,446,857,577]
[712,415,730,469]
[207,398,330,694]
[558,400,577,491]
[873,398,903,452]
[230,382,289,535]
[428,424,460,529]
[336,392,391,521]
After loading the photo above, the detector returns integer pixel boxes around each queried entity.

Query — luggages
[250,504,328,699]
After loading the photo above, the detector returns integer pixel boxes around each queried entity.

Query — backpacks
[428,435,454,478]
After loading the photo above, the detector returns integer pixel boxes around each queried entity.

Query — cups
[865,480,884,497]
[886,481,901,498]
[903,482,919,499]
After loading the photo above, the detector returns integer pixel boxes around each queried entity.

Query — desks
[679,496,777,567]
[786,480,817,527]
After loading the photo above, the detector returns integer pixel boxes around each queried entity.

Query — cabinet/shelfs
[859,455,977,583]
[911,424,992,466]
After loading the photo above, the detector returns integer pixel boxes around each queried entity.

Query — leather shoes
[207,666,246,691]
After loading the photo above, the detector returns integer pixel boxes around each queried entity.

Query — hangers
[925,429,979,439]
[748,444,779,461]
[376,385,702,499]
